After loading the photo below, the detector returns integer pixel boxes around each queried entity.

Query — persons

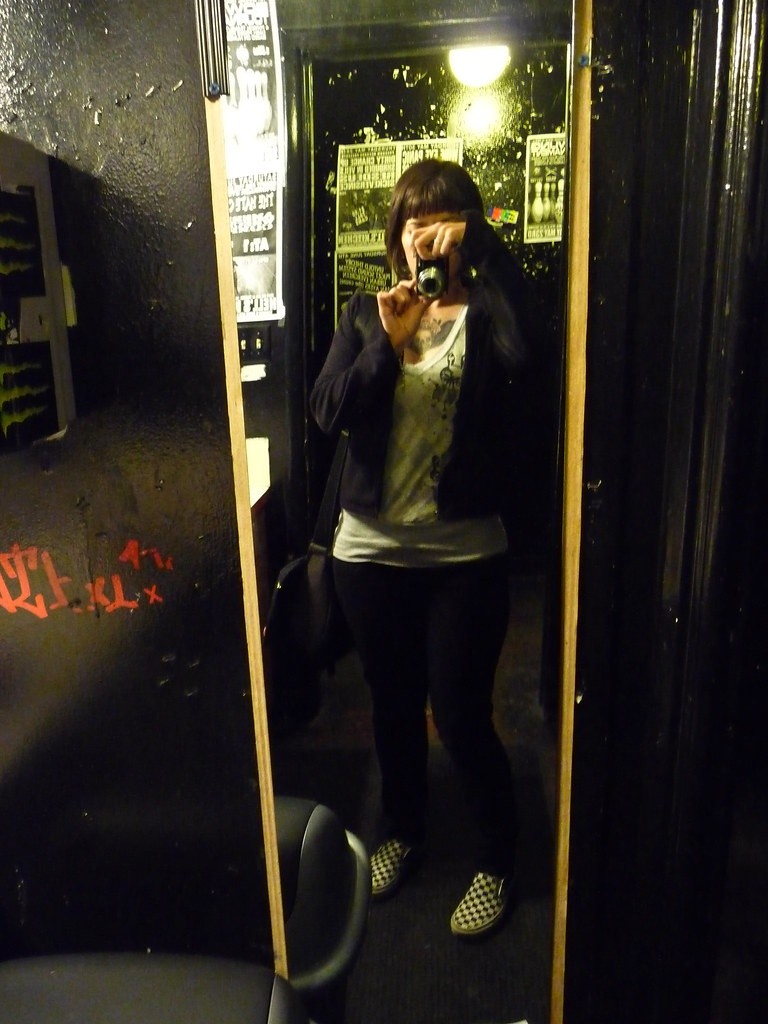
[290,160,537,942]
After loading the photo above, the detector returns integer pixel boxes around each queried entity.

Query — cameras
[415,253,449,298]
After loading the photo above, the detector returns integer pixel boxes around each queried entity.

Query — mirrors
[192,0,594,1024]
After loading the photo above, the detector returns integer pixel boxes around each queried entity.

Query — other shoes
[368,837,415,893]
[449,870,511,934]
